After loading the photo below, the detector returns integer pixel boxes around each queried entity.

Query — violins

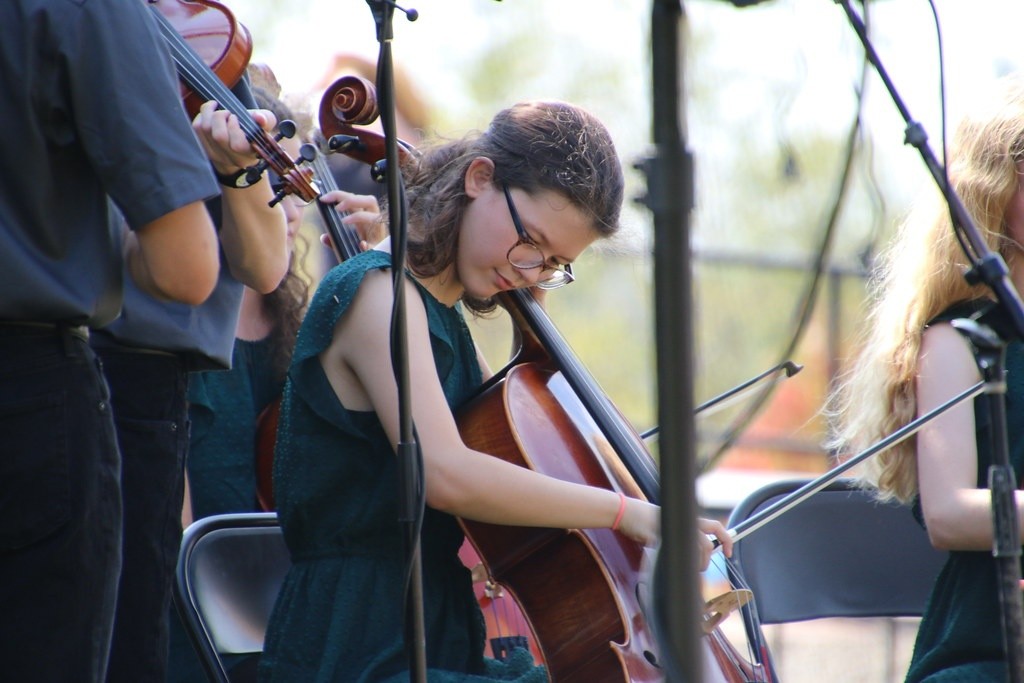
[151,0,325,210]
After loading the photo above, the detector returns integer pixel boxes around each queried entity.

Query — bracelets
[611,493,626,531]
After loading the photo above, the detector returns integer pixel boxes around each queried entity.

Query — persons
[257,100,732,683]
[795,80,1024,683]
[0,0,387,682]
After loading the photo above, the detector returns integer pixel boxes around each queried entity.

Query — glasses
[502,180,575,290]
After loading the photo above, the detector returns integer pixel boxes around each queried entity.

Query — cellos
[317,71,771,681]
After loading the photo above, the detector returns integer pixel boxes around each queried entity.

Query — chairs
[174,510,289,683]
[726,479,946,683]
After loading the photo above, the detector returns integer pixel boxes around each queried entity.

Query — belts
[0,323,91,343]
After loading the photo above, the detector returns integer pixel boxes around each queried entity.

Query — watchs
[215,158,269,188]
